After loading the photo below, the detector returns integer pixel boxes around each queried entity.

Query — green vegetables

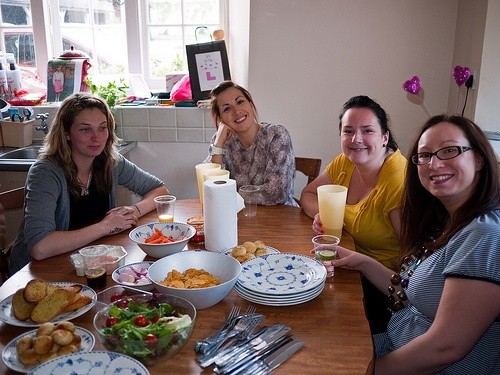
[101,299,192,363]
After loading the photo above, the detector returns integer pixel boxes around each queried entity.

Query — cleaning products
[5,58,20,97]
[0,58,5,97]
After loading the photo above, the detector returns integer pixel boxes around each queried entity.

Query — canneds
[186,217,204,244]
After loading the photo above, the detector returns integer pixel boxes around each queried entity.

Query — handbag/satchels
[170,73,192,102]
[186,26,231,100]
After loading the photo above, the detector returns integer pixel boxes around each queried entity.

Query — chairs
[292,156,322,208]
[0,185,26,286]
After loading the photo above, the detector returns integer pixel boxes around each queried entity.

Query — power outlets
[471,70,478,90]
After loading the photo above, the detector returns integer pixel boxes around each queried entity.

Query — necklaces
[77,173,91,196]
[387,228,446,311]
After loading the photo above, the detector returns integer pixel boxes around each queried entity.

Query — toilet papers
[202,176,246,253]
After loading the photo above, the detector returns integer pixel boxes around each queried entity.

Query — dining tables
[0,198,376,375]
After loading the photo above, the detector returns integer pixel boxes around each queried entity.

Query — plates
[27,350,150,375]
[222,244,327,306]
[0,280,97,328]
[1,326,95,373]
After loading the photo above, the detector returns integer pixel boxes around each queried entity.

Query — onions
[117,264,153,285]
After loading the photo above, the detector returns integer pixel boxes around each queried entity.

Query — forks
[193,304,266,363]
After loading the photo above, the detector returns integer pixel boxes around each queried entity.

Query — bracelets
[135,205,141,215]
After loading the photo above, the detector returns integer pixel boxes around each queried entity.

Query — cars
[0,25,110,81]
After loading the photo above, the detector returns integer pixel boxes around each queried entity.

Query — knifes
[199,323,303,375]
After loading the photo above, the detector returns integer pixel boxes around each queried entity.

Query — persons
[310,114,500,375]
[200,82,300,208]
[300,95,409,333]
[52,65,64,102]
[9,92,170,278]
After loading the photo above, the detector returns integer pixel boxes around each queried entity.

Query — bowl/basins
[92,292,196,366]
[148,248,243,310]
[111,261,157,290]
[128,221,196,258]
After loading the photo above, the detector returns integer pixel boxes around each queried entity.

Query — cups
[154,196,176,222]
[79,245,109,291]
[316,184,348,241]
[195,163,230,219]
[239,185,260,218]
[312,235,341,278]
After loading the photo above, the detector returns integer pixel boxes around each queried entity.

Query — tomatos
[109,335,120,347]
[150,316,159,323]
[135,314,147,326]
[145,334,158,349]
[117,300,128,308]
[110,294,122,302]
[106,317,118,327]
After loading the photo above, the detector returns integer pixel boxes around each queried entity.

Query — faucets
[35,112,49,135]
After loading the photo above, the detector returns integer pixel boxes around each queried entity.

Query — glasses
[412,146,479,164]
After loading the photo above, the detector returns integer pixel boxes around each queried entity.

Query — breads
[17,320,82,363]
[230,240,270,264]
[12,278,94,322]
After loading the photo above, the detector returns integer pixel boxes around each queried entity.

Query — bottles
[6,63,21,96]
[0,62,10,93]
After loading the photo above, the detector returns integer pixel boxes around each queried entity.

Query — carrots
[144,228,180,244]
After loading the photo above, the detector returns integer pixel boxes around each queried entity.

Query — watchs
[209,144,224,155]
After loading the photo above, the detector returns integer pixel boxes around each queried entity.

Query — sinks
[0,145,44,161]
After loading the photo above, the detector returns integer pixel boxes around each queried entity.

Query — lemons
[319,250,334,257]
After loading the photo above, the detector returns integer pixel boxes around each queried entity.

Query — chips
[160,268,224,288]
